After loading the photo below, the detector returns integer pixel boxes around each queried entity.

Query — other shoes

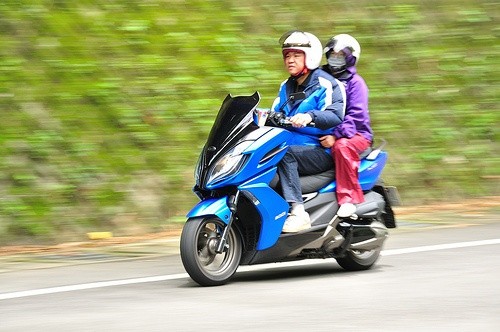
[281,211,312,234]
[337,203,357,217]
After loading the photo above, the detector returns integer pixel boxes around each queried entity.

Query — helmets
[324,34,361,78]
[282,31,323,70]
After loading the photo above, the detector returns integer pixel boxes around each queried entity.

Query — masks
[328,53,346,68]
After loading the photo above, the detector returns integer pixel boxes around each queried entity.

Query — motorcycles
[178,91,401,287]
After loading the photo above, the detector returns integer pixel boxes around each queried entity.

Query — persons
[317,34,375,218]
[254,30,346,234]
